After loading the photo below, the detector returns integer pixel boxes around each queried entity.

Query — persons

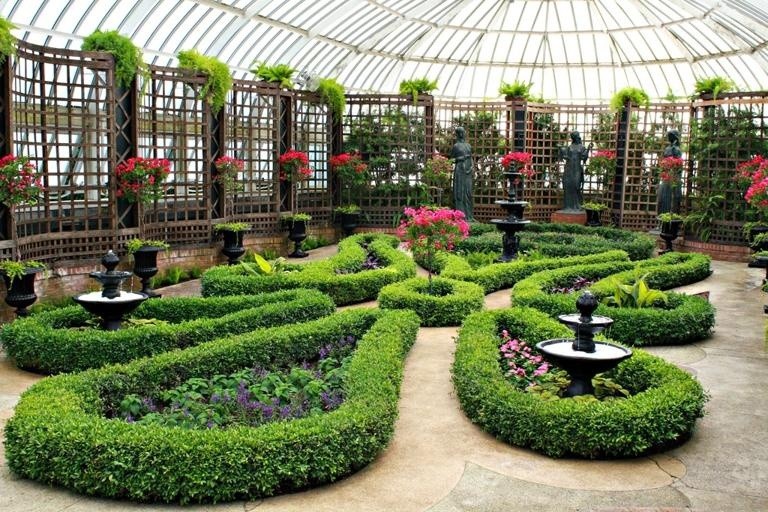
[657,130,682,218]
[561,130,593,211]
[446,126,475,222]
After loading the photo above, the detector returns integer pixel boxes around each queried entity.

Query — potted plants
[1,260,50,319]
[129,200,372,303]
[656,211,691,254]
[742,220,768,316]
[583,201,613,227]
